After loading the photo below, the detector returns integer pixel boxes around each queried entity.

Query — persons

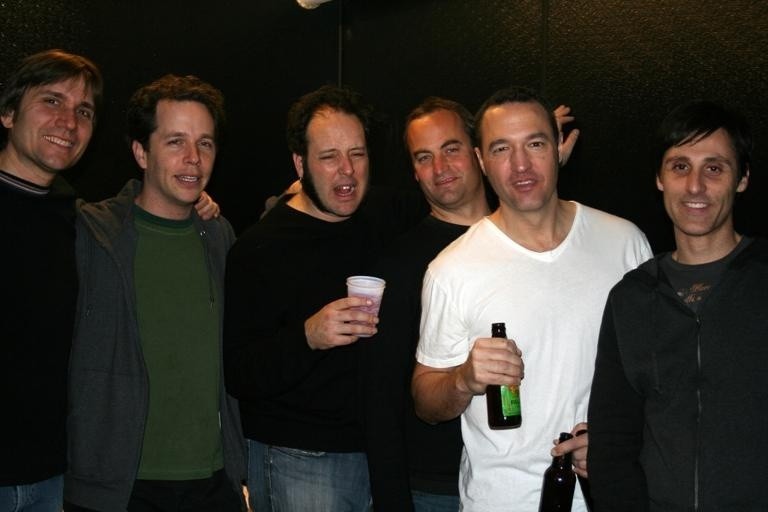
[61,72,248,512]
[363,93,505,512]
[585,102,766,511]
[408,83,661,512]
[221,80,585,512]
[1,47,224,512]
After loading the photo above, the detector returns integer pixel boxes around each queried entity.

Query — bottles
[485,322,522,430]
[538,429,576,512]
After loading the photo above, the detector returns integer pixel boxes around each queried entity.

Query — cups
[344,276,386,339]
